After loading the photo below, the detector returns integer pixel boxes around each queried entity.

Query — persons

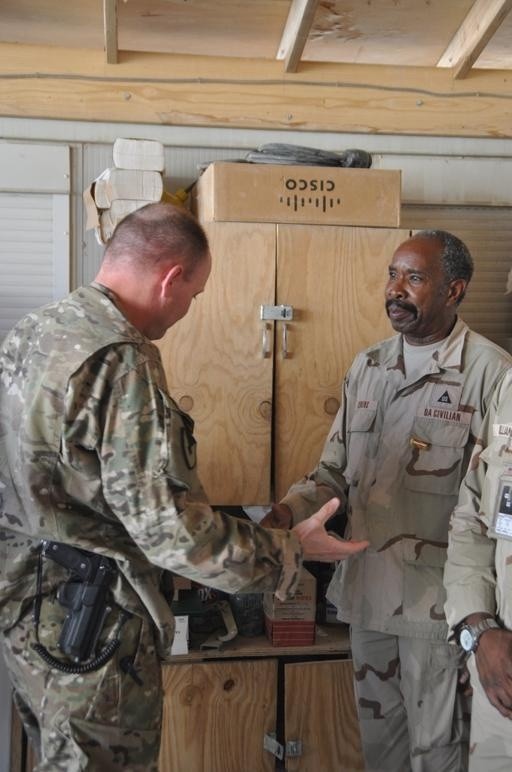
[258,226,510,772]
[442,363,510,772]
[0,201,371,772]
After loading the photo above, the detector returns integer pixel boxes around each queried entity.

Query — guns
[38,539,116,662]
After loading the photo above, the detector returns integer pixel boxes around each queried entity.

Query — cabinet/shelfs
[154,618,367,772]
[148,221,429,506]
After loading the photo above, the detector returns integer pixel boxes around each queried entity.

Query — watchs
[456,618,500,654]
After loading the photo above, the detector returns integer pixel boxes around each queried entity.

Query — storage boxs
[188,160,401,229]
